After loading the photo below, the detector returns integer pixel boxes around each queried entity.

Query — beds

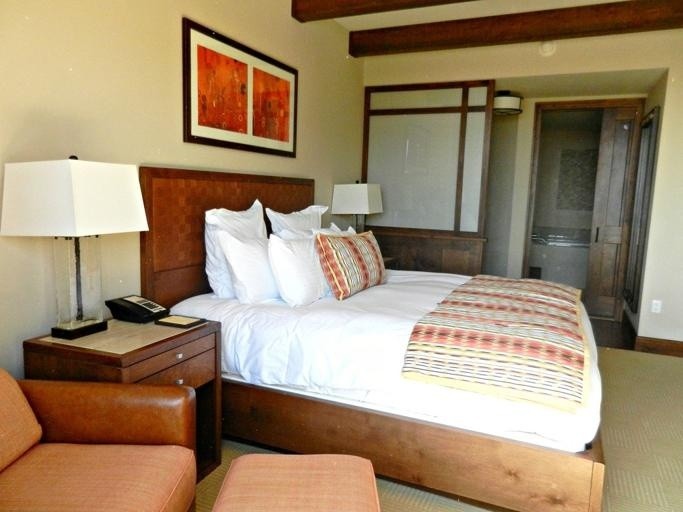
[141,166,606,512]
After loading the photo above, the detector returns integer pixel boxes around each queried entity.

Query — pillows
[317,230,388,302]
[205,199,270,300]
[278,223,344,239]
[266,206,329,234]
[220,231,281,305]
[270,226,358,306]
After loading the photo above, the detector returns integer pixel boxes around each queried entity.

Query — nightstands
[24,311,222,486]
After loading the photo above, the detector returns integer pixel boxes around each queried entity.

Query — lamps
[1,156,150,341]
[331,182,385,233]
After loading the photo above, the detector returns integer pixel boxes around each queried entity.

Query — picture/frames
[184,18,299,159]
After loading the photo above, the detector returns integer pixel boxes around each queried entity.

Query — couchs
[212,455,380,512]
[1,368,199,512]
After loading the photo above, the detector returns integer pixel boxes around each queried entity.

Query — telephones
[104,295,168,324]
